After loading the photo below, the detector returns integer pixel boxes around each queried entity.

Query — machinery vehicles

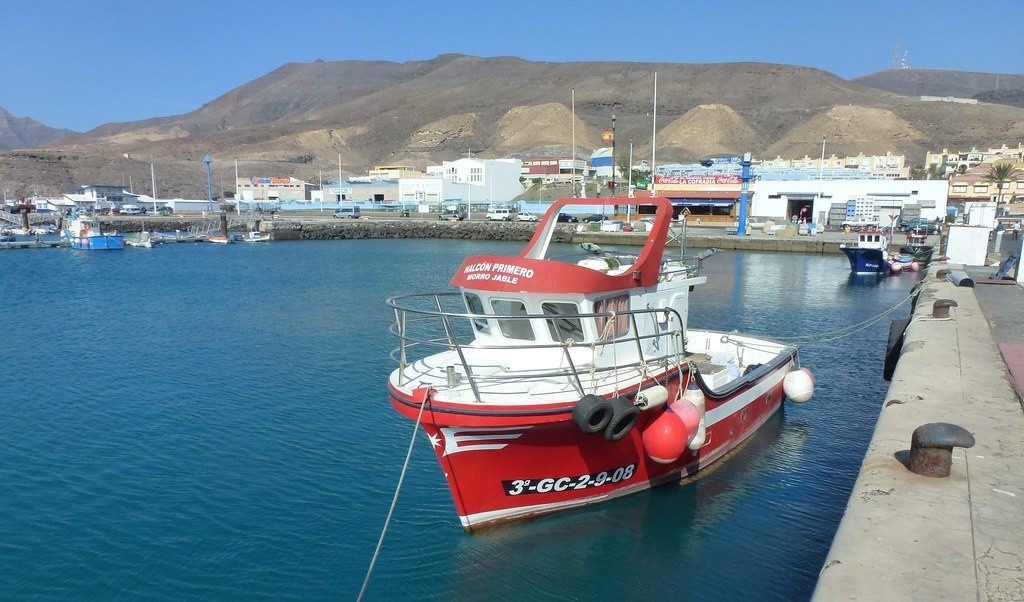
[399,209,410,218]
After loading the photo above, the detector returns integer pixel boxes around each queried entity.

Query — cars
[581,214,609,224]
[557,213,579,223]
[438,210,467,221]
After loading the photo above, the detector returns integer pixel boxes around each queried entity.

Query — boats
[242,232,271,243]
[131,241,152,248]
[380,194,818,536]
[901,233,932,267]
[0,226,56,242]
[838,231,894,277]
[207,235,235,244]
[64,229,125,250]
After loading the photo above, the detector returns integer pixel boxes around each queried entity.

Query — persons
[270,209,275,221]
[996,221,1004,231]
[1011,221,1021,241]
[844,224,851,239]
[797,216,806,226]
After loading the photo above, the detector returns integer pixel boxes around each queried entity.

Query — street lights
[3,190,10,202]
[610,115,617,199]
[886,213,899,251]
[627,138,633,225]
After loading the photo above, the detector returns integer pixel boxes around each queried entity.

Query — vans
[332,206,361,219]
[120,207,140,215]
[517,211,539,222]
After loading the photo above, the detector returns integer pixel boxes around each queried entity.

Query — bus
[995,218,1024,227]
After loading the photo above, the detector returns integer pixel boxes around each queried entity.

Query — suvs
[486,209,513,221]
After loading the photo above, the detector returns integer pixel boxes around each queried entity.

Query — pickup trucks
[746,218,764,229]
[898,217,939,232]
